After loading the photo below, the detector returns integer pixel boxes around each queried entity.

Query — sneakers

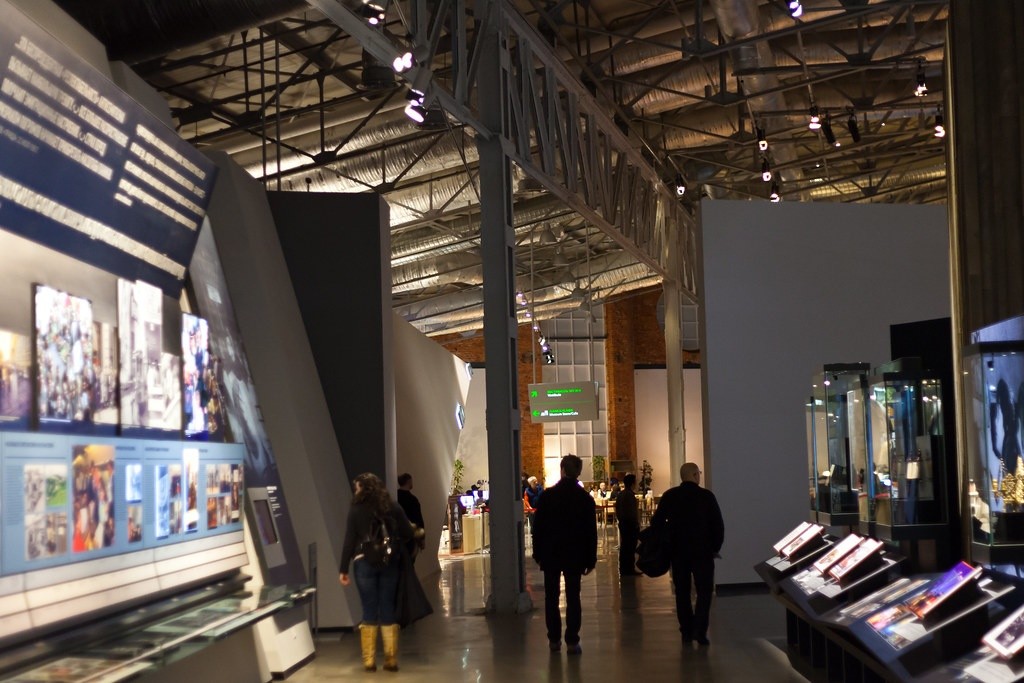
[566,643,582,655]
[549,639,562,651]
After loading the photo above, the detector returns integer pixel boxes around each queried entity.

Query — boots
[357,623,378,672]
[379,623,401,672]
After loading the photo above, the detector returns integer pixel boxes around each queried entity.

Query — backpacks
[361,504,400,565]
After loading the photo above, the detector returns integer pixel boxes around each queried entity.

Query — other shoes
[692,629,710,645]
[620,568,643,576]
[682,631,692,641]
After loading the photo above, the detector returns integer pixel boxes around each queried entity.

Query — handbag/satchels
[636,525,670,577]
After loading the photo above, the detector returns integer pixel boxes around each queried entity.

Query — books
[905,560,981,618]
[773,520,824,557]
[814,534,885,581]
[981,604,1024,660]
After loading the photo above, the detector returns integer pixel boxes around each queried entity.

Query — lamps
[404,45,436,123]
[675,173,686,194]
[518,283,555,364]
[539,220,597,324]
[758,60,945,203]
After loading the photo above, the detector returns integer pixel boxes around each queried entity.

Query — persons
[866,462,882,497]
[460,484,487,515]
[989,378,1024,513]
[397,474,426,581]
[524,476,544,547]
[615,474,644,576]
[158,467,199,536]
[650,463,725,654]
[529,455,598,655]
[209,464,243,530]
[520,472,529,501]
[74,459,115,549]
[340,473,433,673]
[589,479,654,522]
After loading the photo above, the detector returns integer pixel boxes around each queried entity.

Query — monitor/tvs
[460,490,489,507]
[253,499,278,545]
